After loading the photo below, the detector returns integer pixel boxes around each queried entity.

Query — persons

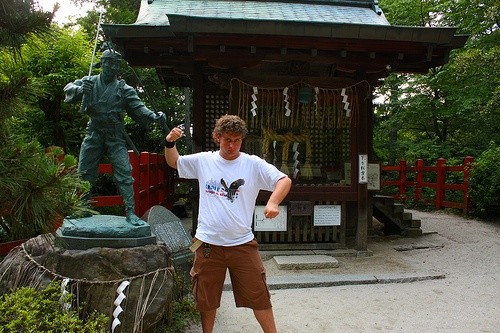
[63,49,166,226]
[165,115,292,333]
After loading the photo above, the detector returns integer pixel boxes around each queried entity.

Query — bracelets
[165,137,175,148]
